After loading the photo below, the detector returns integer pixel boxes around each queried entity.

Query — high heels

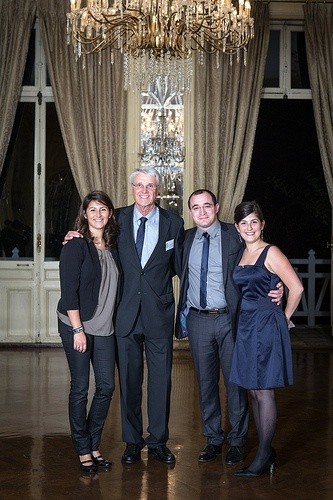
[92,455,113,467]
[78,455,96,473]
[235,448,277,477]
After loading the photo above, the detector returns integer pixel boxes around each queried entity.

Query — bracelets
[72,324,84,334]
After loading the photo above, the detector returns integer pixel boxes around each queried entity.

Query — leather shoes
[225,447,245,464]
[199,444,222,462]
[148,445,175,464]
[121,445,141,464]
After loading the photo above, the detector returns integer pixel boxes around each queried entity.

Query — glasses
[132,183,158,190]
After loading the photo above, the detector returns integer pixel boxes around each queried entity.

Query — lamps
[65,0,254,96]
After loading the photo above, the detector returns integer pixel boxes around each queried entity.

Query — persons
[62,168,185,464]
[229,200,304,477]
[173,189,284,463]
[56,191,122,473]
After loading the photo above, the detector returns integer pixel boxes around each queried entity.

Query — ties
[136,217,149,266]
[200,233,210,309]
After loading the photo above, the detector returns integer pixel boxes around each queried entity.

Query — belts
[190,307,228,314]
